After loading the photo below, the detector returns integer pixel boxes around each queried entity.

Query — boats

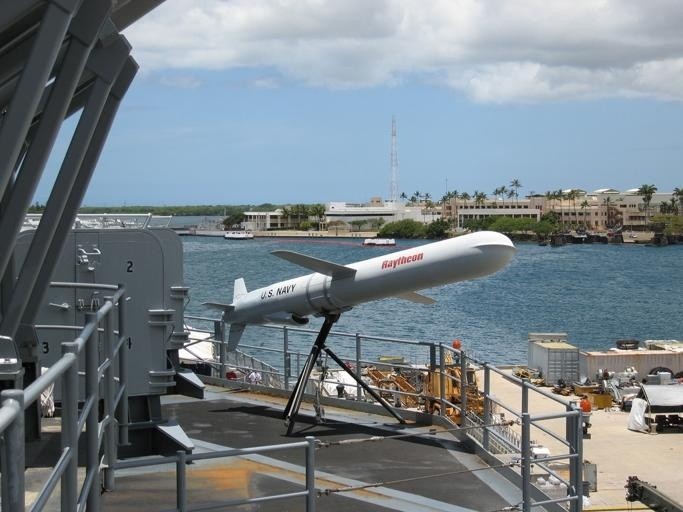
[223,233,254,240]
[362,237,395,246]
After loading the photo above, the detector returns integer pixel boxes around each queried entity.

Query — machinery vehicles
[366,336,494,424]
